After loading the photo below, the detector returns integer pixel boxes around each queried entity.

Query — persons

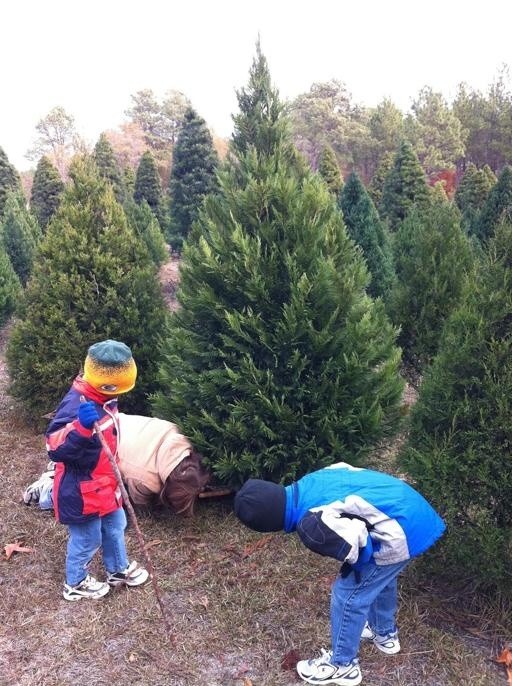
[22,409,211,517]
[42,338,151,602]
[232,458,447,686]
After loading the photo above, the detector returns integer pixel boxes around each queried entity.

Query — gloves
[77,402,100,429]
[338,563,361,585]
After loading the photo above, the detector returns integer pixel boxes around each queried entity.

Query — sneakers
[295,650,362,686]
[359,620,402,655]
[63,577,111,603]
[23,472,54,506]
[108,562,150,587]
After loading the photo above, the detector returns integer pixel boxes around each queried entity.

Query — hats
[232,478,287,533]
[82,340,137,396]
